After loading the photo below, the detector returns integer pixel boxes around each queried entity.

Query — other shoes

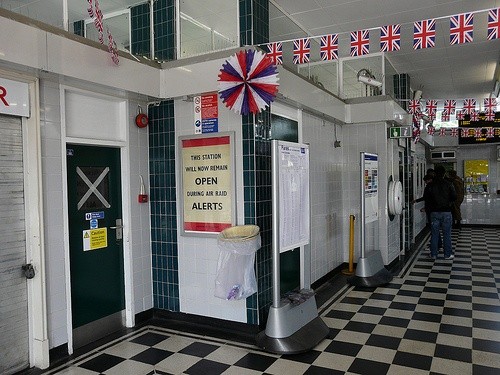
[428,253,436,260]
[445,254,454,260]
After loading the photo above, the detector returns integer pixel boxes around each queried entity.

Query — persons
[410,168,465,250]
[423,166,457,259]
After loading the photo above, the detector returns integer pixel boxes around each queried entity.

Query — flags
[426,100,437,115]
[487,8,500,40]
[380,25,400,52]
[87,0,120,64]
[321,34,338,61]
[412,112,495,143]
[413,20,436,49]
[449,13,474,45]
[443,99,455,114]
[484,98,497,112]
[409,99,420,114]
[293,38,310,64]
[463,98,476,114]
[266,42,283,65]
[350,30,369,57]
